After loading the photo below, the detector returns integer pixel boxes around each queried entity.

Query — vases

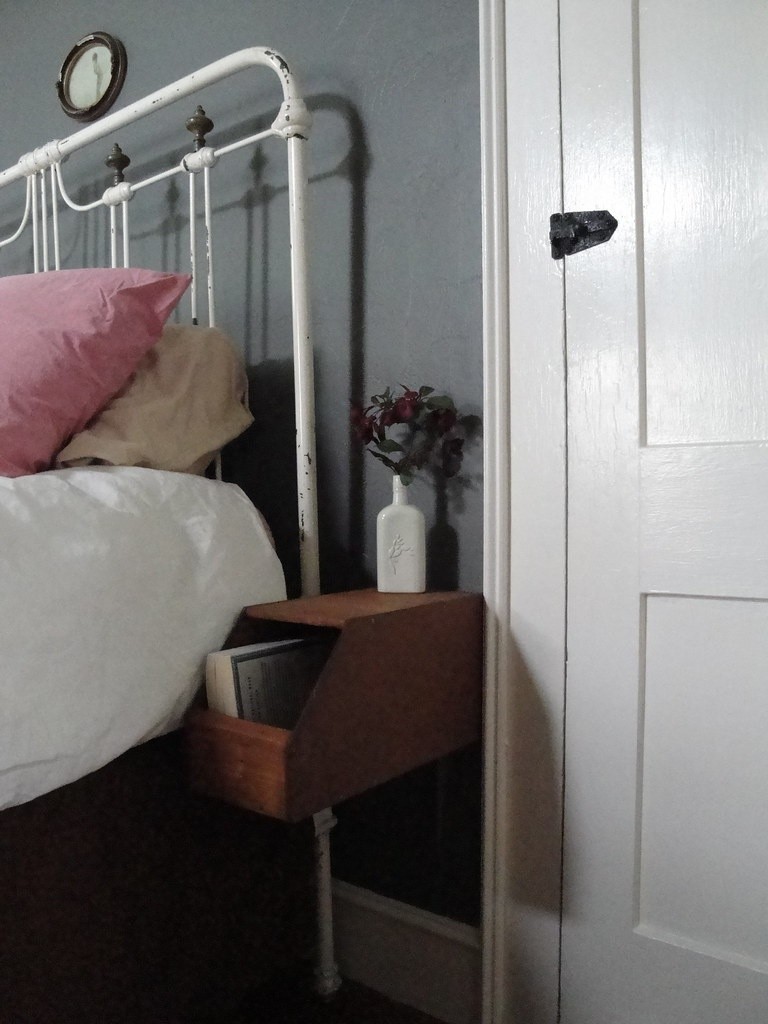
[377,475,427,592]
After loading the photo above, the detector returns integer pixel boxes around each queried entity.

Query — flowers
[348,381,482,485]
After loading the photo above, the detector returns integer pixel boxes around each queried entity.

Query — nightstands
[183,588,483,823]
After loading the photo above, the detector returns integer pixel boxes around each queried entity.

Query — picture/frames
[56,32,128,122]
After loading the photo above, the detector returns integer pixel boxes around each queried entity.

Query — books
[207,634,335,731]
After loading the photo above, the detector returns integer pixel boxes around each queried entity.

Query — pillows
[0,268,193,478]
[55,326,255,472]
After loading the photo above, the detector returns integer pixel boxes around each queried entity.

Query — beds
[0,47,339,1024]
